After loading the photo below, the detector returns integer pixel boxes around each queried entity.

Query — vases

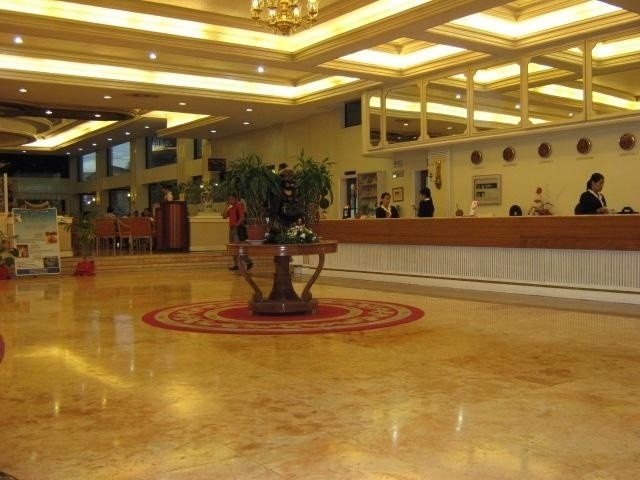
[535,209,549,214]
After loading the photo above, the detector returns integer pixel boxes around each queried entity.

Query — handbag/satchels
[236,224,248,241]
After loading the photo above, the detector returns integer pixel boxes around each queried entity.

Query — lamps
[248,0,319,36]
[127,191,136,204]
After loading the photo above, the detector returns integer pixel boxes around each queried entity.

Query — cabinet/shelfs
[357,171,388,218]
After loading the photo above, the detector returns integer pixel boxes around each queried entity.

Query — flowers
[285,224,318,241]
[528,187,553,216]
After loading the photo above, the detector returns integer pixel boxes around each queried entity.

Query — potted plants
[197,148,295,243]
[59,205,104,276]
[0,229,20,281]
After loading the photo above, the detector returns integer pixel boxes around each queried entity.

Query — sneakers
[229,265,239,270]
[246,262,254,271]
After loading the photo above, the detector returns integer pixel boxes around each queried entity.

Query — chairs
[87,216,155,256]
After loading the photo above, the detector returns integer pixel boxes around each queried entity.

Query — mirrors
[362,19,639,153]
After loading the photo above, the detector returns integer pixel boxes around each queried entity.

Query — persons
[376,193,399,219]
[574,173,608,213]
[223,193,254,269]
[81,184,173,222]
[418,188,436,217]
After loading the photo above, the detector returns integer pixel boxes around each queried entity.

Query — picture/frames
[391,187,404,202]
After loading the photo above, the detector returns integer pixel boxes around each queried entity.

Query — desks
[224,240,338,314]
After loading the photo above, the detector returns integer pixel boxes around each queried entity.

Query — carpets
[140,298,424,337]
